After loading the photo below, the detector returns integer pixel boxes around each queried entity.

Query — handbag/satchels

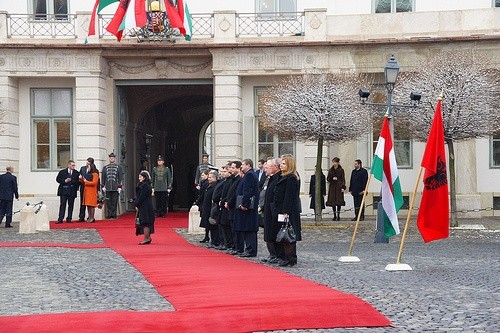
[135,218,141,225]
[275,217,296,244]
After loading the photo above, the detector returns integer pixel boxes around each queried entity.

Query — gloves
[102,187,107,192]
[117,188,122,193]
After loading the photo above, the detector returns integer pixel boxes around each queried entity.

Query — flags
[369,118,404,239]
[418,100,449,243]
[84,0,192,45]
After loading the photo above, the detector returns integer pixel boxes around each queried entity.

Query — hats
[207,165,219,171]
[109,153,115,157]
[157,155,164,161]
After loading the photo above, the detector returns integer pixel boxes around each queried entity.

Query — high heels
[86,218,95,223]
[199,237,210,243]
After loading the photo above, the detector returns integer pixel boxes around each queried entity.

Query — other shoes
[5,225,13,228]
[261,257,289,266]
[76,219,85,222]
[137,238,152,245]
[337,217,340,221]
[351,216,357,221]
[67,220,71,223]
[207,243,256,257]
[332,218,337,221]
[55,221,63,224]
[360,216,364,221]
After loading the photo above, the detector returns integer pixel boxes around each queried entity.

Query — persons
[128,172,155,244]
[139,156,149,172]
[326,157,346,221]
[75,157,100,223]
[56,0,67,20]
[309,165,326,223]
[0,165,20,228]
[349,159,368,221]
[152,154,173,217]
[255,154,302,267]
[55,160,81,224]
[196,158,260,257]
[195,154,214,198]
[100,152,122,220]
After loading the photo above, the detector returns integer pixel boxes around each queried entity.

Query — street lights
[357,53,424,244]
